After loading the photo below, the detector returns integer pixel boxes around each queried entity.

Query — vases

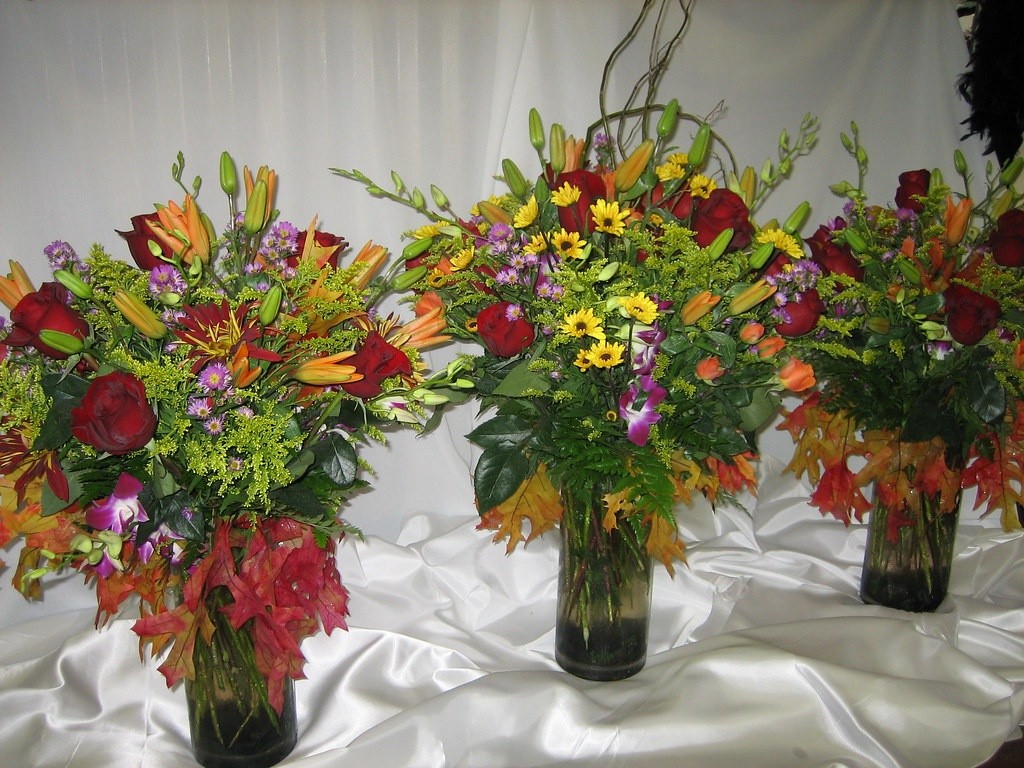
[554,464,654,683]
[854,437,964,612]
[181,590,297,768]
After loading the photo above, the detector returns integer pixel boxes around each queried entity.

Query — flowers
[770,120,1024,544]
[329,100,820,578]
[0,152,477,716]
